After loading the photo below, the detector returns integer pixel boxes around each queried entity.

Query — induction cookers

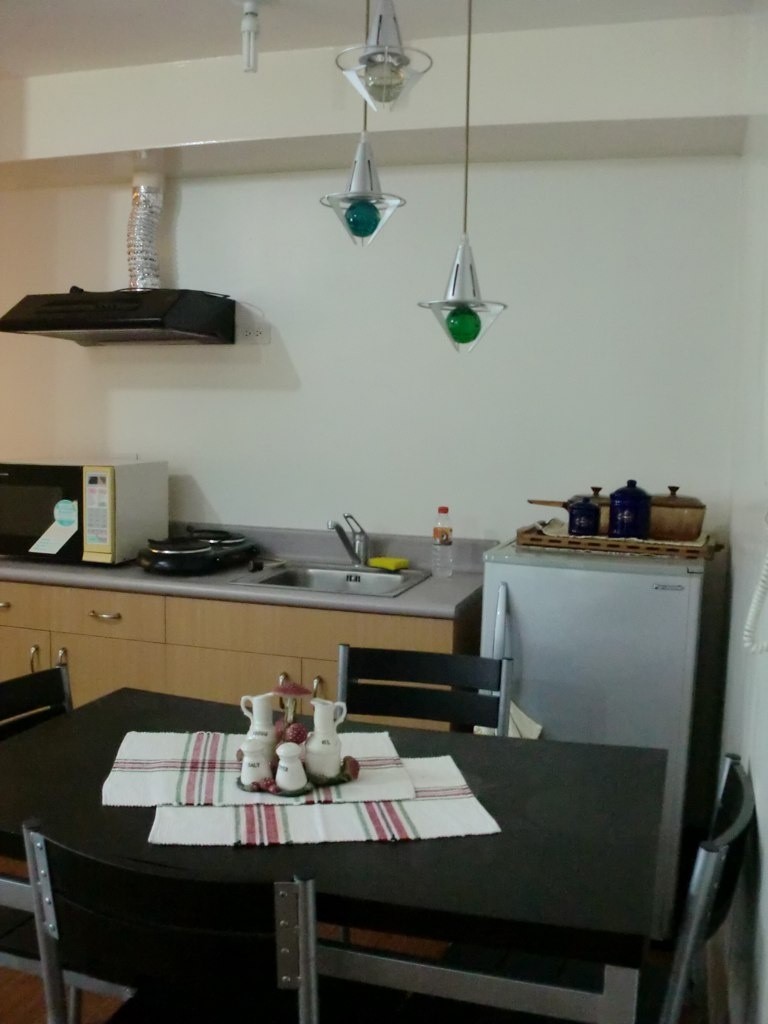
[1,454,170,569]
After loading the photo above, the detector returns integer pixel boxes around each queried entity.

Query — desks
[1,687,667,1024]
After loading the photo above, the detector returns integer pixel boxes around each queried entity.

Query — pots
[650,486,707,541]
[527,487,609,536]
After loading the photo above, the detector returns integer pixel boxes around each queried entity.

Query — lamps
[320,0,407,248]
[336,0,432,114]
[418,0,508,353]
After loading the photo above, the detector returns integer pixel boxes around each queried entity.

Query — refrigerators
[471,538,707,942]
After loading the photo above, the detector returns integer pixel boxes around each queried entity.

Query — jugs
[304,698,347,778]
[240,692,278,761]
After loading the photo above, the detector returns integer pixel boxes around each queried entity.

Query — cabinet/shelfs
[166,596,482,961]
[0,582,166,880]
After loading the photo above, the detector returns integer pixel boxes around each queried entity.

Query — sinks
[254,567,414,595]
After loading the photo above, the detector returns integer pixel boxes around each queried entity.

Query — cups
[568,498,600,536]
[609,479,651,540]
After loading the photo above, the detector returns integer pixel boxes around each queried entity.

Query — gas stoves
[136,532,258,576]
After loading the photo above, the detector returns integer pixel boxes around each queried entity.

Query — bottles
[240,739,273,786]
[432,506,453,579]
[275,742,308,790]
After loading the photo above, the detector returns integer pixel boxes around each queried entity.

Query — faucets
[326,512,370,564]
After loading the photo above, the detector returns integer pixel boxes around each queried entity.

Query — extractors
[1,169,236,347]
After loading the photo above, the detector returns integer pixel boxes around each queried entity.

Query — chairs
[2,643,754,1023]
